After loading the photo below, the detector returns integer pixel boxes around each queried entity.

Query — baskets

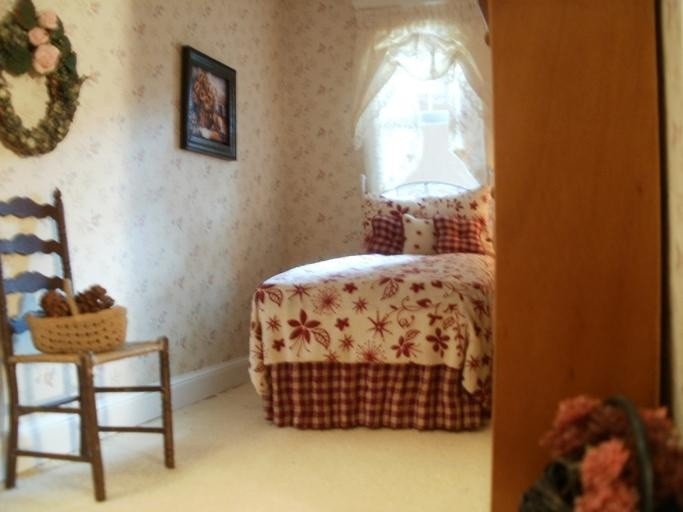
[27,278,127,354]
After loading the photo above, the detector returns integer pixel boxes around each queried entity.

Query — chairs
[0,188,175,501]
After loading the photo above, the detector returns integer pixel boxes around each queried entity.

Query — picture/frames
[181,43,237,160]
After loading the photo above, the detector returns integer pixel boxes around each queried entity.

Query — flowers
[520,393,683,511]
[0,0,81,158]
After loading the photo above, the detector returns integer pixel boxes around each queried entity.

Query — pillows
[433,217,488,254]
[401,213,435,254]
[367,214,405,254]
[360,185,495,255]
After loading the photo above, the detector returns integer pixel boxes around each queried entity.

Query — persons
[191,70,228,142]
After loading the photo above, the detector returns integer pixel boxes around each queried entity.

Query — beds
[249,254,495,431]
